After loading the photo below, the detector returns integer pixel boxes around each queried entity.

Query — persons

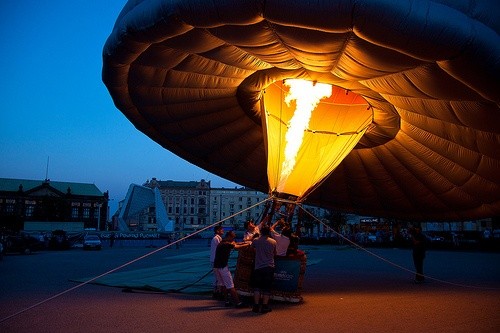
[271,217,291,256]
[286,234,307,270]
[209,224,225,300]
[168,230,189,249]
[244,216,271,247]
[409,223,428,284]
[483,227,492,240]
[249,224,277,313]
[494,226,500,240]
[109,231,115,249]
[214,230,253,309]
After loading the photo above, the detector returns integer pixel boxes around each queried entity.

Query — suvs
[354,232,376,244]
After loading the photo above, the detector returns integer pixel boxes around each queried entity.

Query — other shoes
[261,306,272,313]
[212,292,229,300]
[225,300,233,307]
[416,277,428,283]
[253,305,259,313]
[235,302,248,308]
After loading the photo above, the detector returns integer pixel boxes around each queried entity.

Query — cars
[84,234,102,249]
[421,230,485,249]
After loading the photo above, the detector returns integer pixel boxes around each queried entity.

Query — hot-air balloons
[100,0,500,302]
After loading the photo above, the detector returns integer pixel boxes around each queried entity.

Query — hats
[214,222,222,232]
[243,218,254,228]
